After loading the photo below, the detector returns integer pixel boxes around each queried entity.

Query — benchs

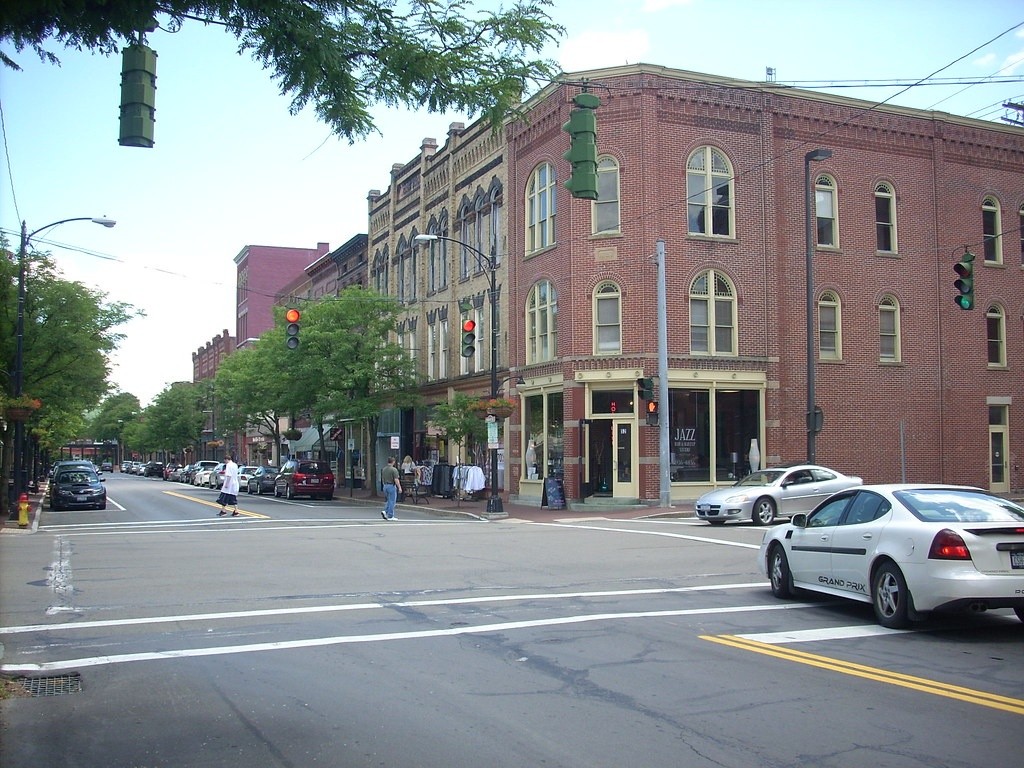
[400,480,430,505]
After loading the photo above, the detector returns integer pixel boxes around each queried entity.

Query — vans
[189,460,218,485]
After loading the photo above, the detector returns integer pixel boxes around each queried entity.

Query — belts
[384,483,394,484]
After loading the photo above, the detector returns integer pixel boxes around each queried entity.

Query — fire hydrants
[17,492,33,528]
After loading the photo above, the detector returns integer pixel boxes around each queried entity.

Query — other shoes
[232,510,239,516]
[216,510,227,517]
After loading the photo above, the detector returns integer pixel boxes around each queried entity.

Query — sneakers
[381,510,387,520]
[387,517,398,521]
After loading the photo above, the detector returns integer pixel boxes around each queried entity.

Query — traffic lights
[286,309,301,349]
[645,401,660,425]
[462,319,476,357]
[562,108,600,200]
[953,262,974,311]
[636,378,654,402]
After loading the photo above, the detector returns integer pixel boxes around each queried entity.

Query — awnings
[293,424,338,452]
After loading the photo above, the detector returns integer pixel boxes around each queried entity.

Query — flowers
[1,395,41,409]
[468,397,518,411]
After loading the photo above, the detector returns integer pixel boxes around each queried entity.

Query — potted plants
[495,407,513,418]
[31,428,47,440]
[282,429,302,440]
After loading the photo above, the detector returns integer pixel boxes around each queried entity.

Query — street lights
[415,234,527,512]
[193,379,215,461]
[8,214,117,521]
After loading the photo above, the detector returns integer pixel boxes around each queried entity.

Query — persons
[78,474,90,482]
[381,458,402,521]
[401,456,416,492]
[216,454,240,517]
[765,473,777,482]
[788,475,795,483]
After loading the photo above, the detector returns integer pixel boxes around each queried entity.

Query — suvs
[273,459,335,501]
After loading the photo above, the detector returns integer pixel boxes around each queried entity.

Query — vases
[7,408,34,421]
[477,411,487,419]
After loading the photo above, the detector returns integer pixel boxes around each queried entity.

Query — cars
[247,466,282,495]
[761,483,1024,631]
[50,468,107,511]
[694,465,863,526]
[48,460,102,479]
[101,463,113,473]
[208,463,242,489]
[120,460,194,484]
[238,466,259,492]
[194,467,214,487]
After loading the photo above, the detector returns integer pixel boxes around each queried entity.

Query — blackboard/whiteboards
[541,477,566,507]
